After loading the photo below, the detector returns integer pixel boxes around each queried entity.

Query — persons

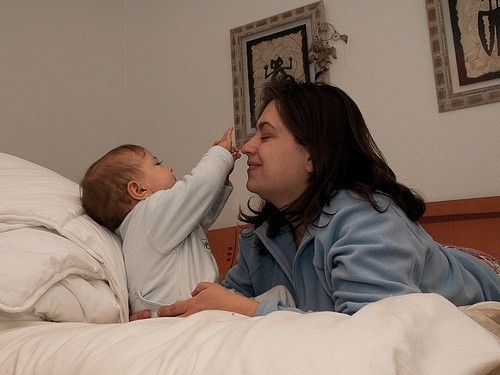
[124,82,500,321]
[81,127,296,318]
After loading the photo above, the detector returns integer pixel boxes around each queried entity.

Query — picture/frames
[230,0,331,150]
[425,0,500,114]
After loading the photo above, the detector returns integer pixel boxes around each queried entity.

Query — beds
[0,151,500,375]
